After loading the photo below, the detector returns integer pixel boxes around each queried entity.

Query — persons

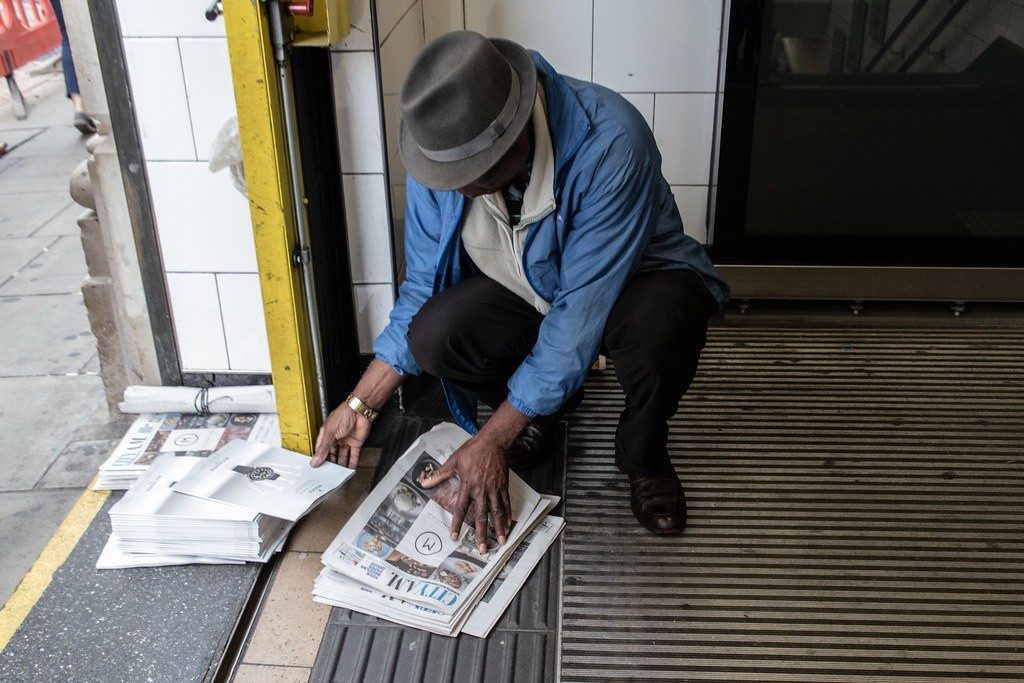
[311,30,733,554]
[50,0,97,135]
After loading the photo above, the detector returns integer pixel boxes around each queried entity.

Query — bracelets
[346,392,378,422]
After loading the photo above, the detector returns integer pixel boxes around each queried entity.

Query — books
[91,413,567,638]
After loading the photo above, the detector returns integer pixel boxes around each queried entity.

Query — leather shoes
[615,438,687,537]
[504,382,584,472]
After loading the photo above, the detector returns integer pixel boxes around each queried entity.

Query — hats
[399,30,536,191]
